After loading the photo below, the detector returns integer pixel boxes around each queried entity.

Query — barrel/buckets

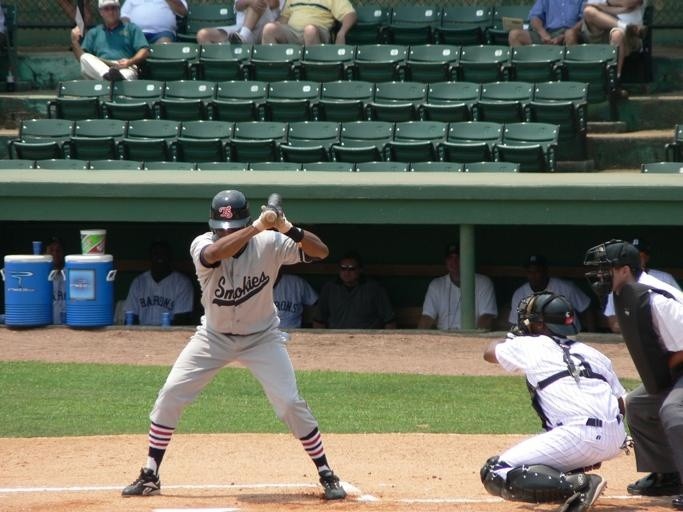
[61,253,118,328]
[0,254,59,327]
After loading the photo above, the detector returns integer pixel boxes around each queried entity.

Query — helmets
[208,190,251,229]
[584,239,640,297]
[517,291,581,336]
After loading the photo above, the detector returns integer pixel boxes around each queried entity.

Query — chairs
[1,2,683,176]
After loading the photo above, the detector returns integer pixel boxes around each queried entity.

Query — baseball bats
[262,193,280,227]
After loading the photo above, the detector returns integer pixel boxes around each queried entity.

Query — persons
[509,255,598,332]
[580,0,648,97]
[272,267,319,328]
[584,239,683,509]
[312,250,397,329]
[418,239,498,331]
[480,293,635,512]
[45,234,73,324]
[604,238,683,333]
[508,0,588,48]
[123,242,194,326]
[122,190,346,500]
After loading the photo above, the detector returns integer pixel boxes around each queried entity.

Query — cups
[160,311,170,325]
[59,310,66,323]
[31,241,41,253]
[123,310,133,325]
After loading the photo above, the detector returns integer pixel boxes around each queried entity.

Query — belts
[587,414,623,425]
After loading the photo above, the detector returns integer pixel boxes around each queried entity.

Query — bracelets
[283,225,304,242]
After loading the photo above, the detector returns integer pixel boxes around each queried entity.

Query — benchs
[113,258,683,331]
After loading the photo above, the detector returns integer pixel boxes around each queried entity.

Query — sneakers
[229,32,245,43]
[568,474,605,512]
[109,68,123,83]
[627,473,683,508]
[122,468,160,496]
[319,470,345,499]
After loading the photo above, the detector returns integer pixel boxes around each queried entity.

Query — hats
[98,0,120,10]
